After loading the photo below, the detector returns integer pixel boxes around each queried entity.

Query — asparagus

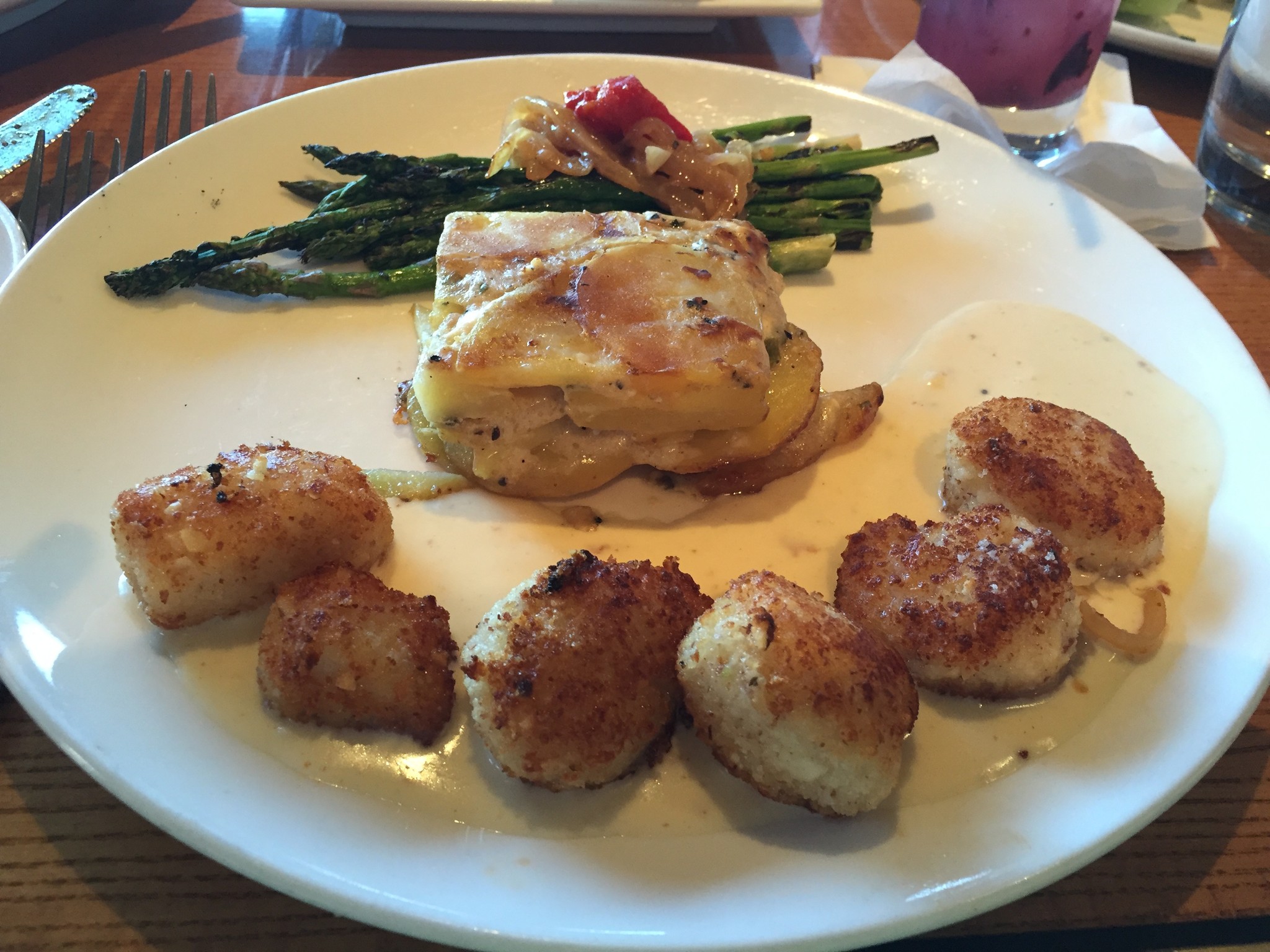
[99,111,938,296]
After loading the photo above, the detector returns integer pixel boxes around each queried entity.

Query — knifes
[0,85,98,178]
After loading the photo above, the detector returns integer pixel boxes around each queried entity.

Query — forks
[18,129,120,254]
[124,69,216,171]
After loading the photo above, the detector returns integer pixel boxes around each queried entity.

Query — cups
[1193,0,1269,237]
[915,0,1119,166]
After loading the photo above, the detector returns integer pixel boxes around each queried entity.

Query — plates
[1108,19,1224,67]
[0,54,1270,952]
[0,0,69,33]
[231,0,823,48]
